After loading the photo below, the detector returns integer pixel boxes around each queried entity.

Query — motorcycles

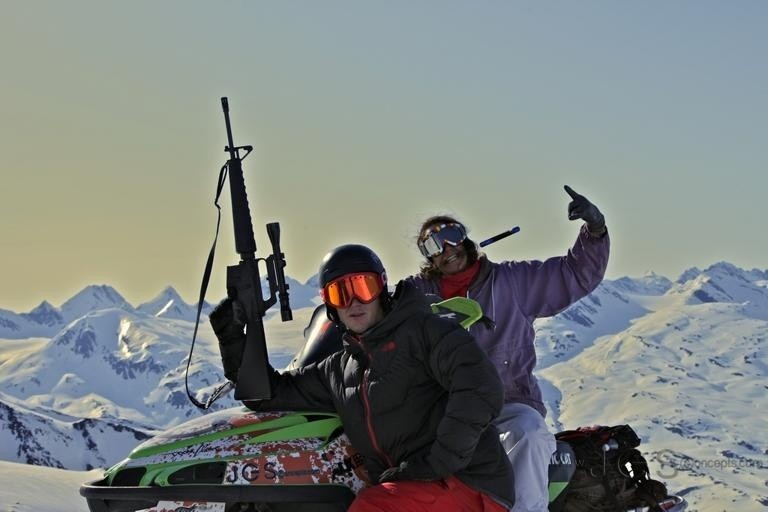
[78,307,690,511]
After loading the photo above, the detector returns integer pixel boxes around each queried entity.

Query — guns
[221,97,293,401]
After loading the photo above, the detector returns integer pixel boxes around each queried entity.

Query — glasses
[320,272,384,310]
[418,223,468,257]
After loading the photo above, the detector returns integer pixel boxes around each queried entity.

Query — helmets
[317,243,386,285]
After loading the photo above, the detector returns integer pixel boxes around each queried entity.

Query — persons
[385,185,610,512]
[208,244,515,512]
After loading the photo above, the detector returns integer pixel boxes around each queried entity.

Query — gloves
[208,287,247,344]
[562,182,606,234]
[377,457,439,487]
